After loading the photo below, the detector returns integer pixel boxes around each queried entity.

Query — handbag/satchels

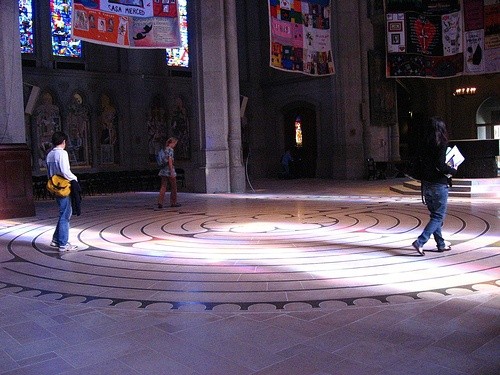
[46,173,71,198]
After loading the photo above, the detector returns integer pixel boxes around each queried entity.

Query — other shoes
[59,242,79,252]
[158,204,163,208]
[438,246,451,252]
[412,240,425,255]
[50,238,59,247]
[171,203,181,207]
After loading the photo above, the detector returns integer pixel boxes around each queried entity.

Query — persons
[281,150,294,179]
[157,136,182,208]
[412,116,452,256]
[45,132,79,252]
